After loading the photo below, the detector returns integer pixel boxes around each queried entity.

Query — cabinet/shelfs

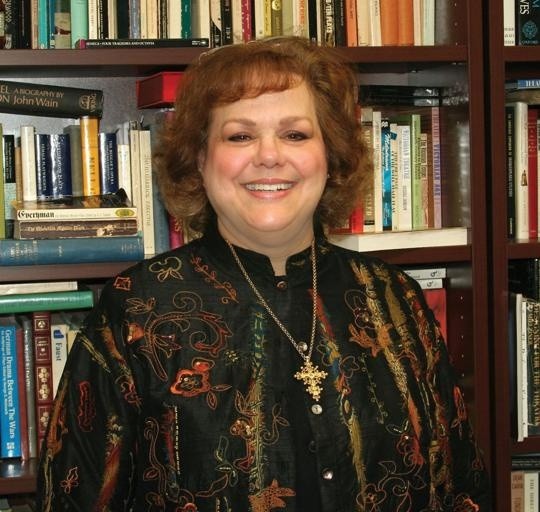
[484,0,539,512]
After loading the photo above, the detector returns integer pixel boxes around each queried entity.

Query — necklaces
[224,237,329,402]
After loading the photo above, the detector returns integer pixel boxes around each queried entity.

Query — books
[1,0,539,49]
[328,101,539,512]
[0,72,206,512]
